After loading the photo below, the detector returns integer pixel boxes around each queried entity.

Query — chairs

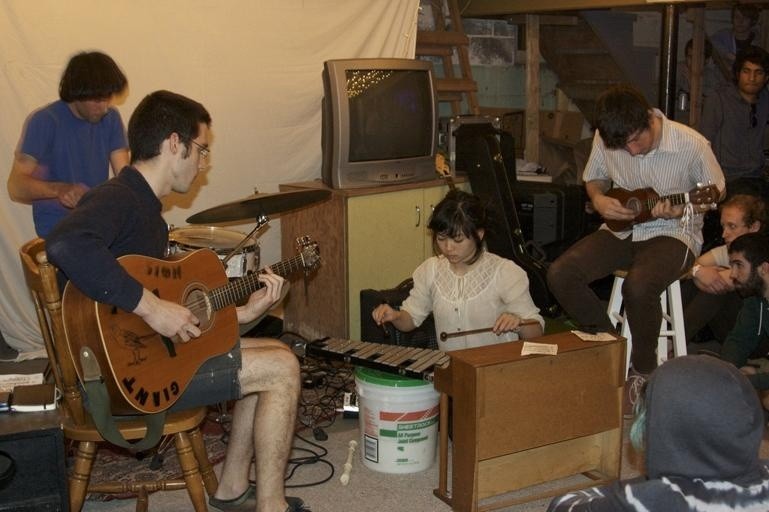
[18,235,226,510]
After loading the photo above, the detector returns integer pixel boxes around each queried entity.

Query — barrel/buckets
[350,363,444,477]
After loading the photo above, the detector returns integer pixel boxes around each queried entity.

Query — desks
[0,356,69,512]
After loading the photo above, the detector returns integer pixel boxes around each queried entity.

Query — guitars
[62,233,322,416]
[605,180,720,231]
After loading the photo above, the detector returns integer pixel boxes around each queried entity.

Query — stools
[608,264,690,378]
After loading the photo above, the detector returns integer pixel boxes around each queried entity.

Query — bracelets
[692,264,702,276]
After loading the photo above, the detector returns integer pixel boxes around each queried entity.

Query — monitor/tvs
[320,56,439,190]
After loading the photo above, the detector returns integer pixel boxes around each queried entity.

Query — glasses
[722,222,747,233]
[189,138,210,158]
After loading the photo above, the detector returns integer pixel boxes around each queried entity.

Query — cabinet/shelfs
[278,179,475,342]
[430,328,630,511]
[488,176,582,249]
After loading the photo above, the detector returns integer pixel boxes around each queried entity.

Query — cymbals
[185,187,332,226]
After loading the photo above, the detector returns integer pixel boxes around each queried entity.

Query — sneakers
[209,485,312,512]
[622,366,650,420]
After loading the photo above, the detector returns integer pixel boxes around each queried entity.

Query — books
[11,384,59,413]
[0,392,11,413]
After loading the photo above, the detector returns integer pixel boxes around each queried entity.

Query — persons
[694,47,767,200]
[469,21,491,35]
[475,38,513,64]
[680,192,769,350]
[714,231,769,410]
[44,85,306,512]
[703,1,765,81]
[674,37,720,126]
[568,138,603,241]
[353,129,394,160]
[538,354,767,512]
[4,47,131,248]
[543,87,730,379]
[369,186,549,360]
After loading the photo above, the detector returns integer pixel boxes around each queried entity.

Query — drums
[168,225,260,282]
